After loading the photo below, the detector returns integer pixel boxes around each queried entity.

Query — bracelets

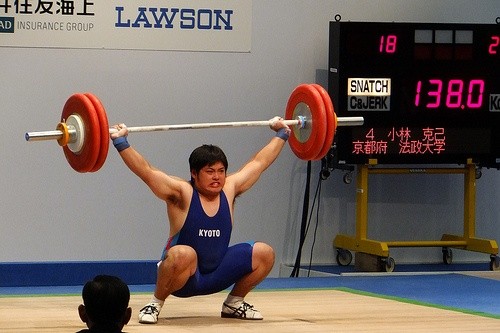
[275,128,290,142]
[111,138,130,151]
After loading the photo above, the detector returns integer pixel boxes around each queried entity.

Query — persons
[73,274,131,333]
[108,116,291,324]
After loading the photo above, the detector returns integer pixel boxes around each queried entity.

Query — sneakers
[221,301,264,320]
[138,303,161,324]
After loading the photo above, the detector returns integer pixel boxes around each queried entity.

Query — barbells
[25,83,365,173]
